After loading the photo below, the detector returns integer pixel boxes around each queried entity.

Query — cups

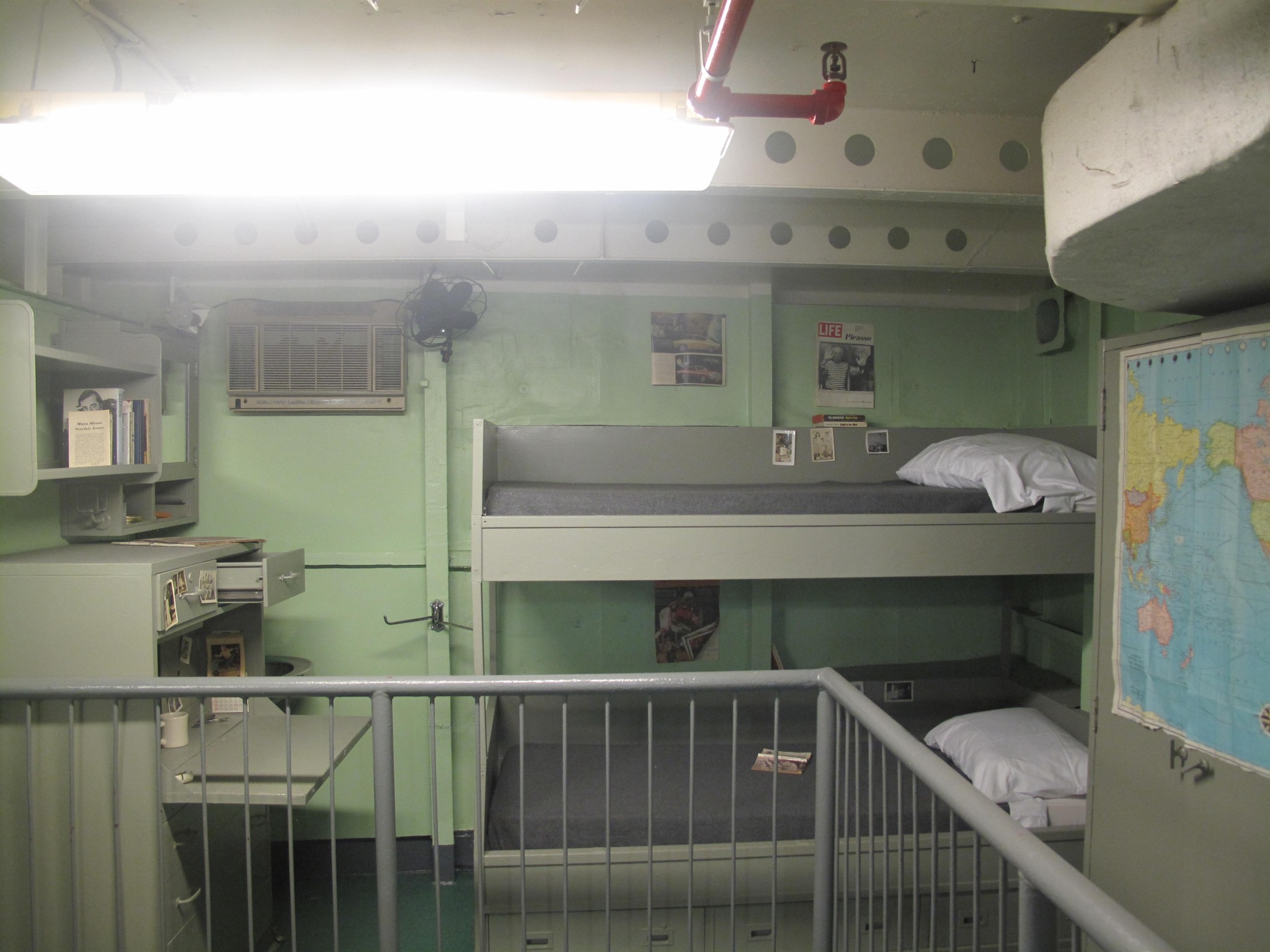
[161,711,189,748]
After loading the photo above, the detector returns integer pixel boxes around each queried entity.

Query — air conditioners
[225,314,404,417]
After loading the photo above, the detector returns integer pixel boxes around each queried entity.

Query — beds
[448,412,1099,903]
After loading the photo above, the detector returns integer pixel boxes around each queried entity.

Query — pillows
[924,705,1088,804]
[894,435,1097,513]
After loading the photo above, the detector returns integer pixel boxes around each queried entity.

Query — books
[60,384,147,466]
[751,748,811,774]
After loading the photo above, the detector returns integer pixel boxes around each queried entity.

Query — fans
[394,278,487,361]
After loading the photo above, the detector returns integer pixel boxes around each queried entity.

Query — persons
[870,440,888,452]
[172,574,185,593]
[819,345,873,391]
[888,683,907,699]
[212,645,237,671]
[776,436,791,456]
[202,575,214,600]
[813,431,831,460]
[655,592,713,662]
[63,389,105,447]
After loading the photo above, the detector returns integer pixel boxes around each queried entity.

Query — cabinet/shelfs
[4,308,371,952]
